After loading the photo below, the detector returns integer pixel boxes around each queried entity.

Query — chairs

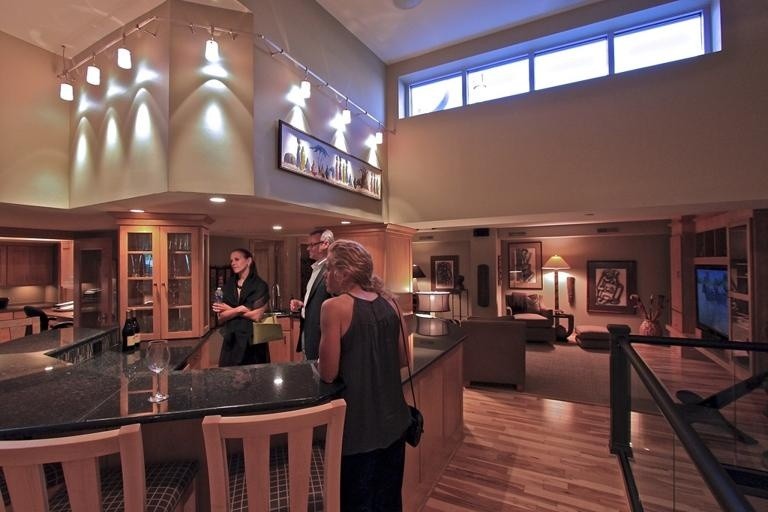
[462,317,525,392]
[505,291,553,342]
[0,306,73,342]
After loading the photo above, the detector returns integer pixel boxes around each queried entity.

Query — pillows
[512,292,540,313]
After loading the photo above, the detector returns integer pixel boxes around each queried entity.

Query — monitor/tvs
[694,264,729,341]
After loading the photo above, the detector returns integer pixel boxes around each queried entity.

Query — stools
[575,325,610,349]
[607,324,631,337]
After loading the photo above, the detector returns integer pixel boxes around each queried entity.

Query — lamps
[300,65,383,144]
[417,320,450,337]
[542,253,571,313]
[414,291,451,319]
[205,31,220,63]
[412,264,426,290]
[60,37,131,101]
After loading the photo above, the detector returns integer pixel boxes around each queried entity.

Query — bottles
[215,287,225,304]
[294,142,380,195]
[123,351,143,380]
[122,307,141,354]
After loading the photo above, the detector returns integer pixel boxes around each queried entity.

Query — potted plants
[630,291,671,337]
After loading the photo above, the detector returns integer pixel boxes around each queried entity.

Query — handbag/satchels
[405,405,423,448]
[252,322,283,344]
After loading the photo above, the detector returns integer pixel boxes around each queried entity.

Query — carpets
[464,334,662,416]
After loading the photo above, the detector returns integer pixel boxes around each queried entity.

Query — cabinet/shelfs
[120,228,197,339]
[693,225,728,258]
[726,217,768,387]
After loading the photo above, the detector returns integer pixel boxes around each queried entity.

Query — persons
[287,228,337,360]
[210,246,272,369]
[314,238,415,512]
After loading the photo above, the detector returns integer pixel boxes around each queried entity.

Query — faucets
[267,280,282,313]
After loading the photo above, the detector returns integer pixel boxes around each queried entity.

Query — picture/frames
[431,251,460,294]
[276,120,384,201]
[586,259,637,314]
[507,241,542,291]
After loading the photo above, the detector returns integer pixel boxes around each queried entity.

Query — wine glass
[142,340,172,403]
